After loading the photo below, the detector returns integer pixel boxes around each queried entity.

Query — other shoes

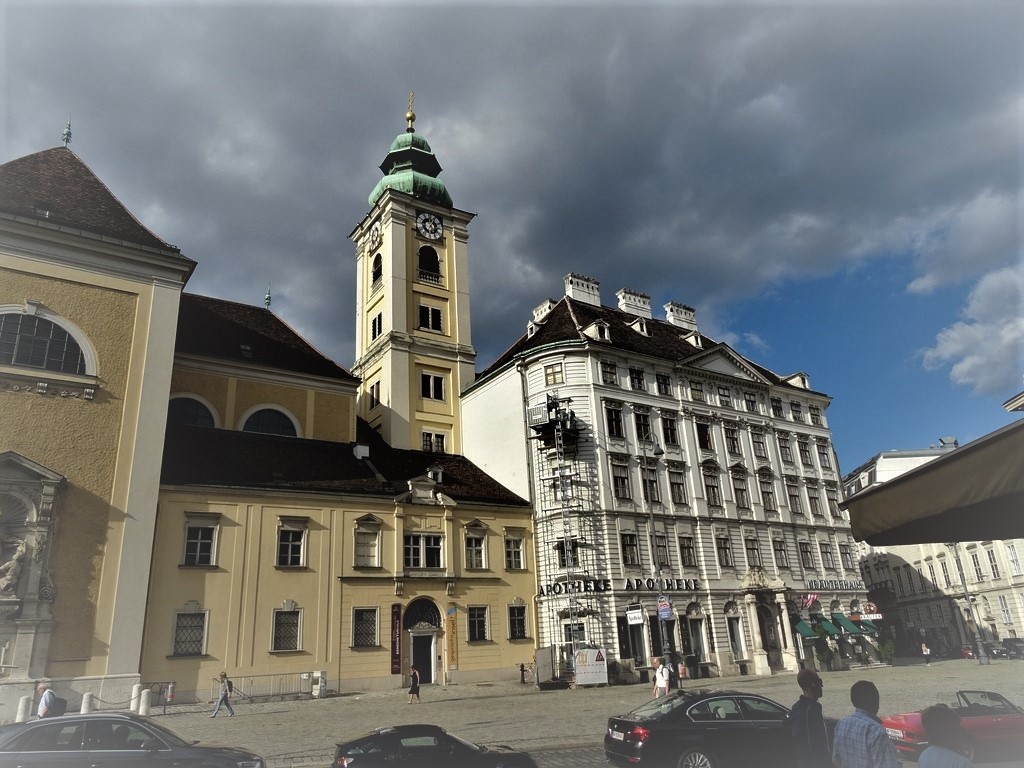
[229,714,233,716]
[408,701,411,704]
[209,715,215,718]
[416,701,419,704]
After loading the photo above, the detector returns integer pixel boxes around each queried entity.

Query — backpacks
[228,680,233,692]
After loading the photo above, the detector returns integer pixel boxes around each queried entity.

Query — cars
[602,689,845,768]
[327,722,537,768]
[1,711,266,768]
[957,637,1024,662]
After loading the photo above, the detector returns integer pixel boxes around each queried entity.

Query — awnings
[787,611,881,638]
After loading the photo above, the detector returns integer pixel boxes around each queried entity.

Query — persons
[921,640,932,666]
[36,682,57,720]
[0,550,25,592]
[652,658,670,699]
[788,669,829,767]
[831,680,899,767]
[918,705,975,768]
[407,664,421,705]
[208,671,235,717]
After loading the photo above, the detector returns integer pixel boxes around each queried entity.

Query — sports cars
[877,689,1024,765]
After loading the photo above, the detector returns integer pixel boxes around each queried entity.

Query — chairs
[68,724,130,747]
[713,706,726,720]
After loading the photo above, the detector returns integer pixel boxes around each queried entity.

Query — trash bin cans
[312,671,327,698]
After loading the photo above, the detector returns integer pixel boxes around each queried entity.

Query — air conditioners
[526,403,549,431]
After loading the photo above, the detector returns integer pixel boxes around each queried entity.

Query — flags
[802,593,819,608]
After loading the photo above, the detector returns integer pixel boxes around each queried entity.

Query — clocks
[369,221,381,250]
[416,212,443,240]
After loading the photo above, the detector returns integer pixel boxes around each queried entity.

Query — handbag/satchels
[927,649,931,654]
[44,690,67,717]
[661,667,679,689]
